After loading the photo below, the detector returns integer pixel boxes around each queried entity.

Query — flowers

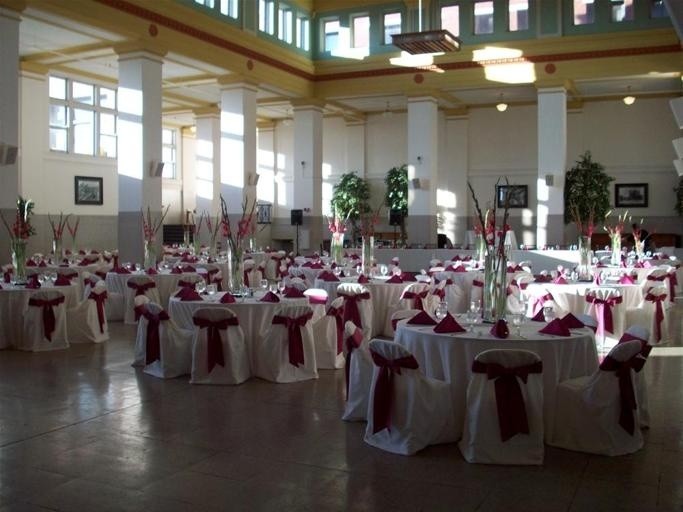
[140,199,170,238]
[322,201,354,233]
[625,214,648,237]
[568,200,600,237]
[48,210,81,240]
[466,175,512,248]
[353,198,385,235]
[602,208,628,234]
[174,193,260,247]
[3,192,38,239]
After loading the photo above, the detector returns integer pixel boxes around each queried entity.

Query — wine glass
[120,259,184,275]
[258,243,416,280]
[445,240,656,281]
[173,240,228,265]
[1,271,59,290]
[433,298,555,339]
[193,279,290,302]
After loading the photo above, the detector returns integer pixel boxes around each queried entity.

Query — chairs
[0,248,676,465]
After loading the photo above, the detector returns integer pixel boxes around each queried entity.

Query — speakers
[290,210,302,226]
[387,209,402,226]
[545,174,554,187]
[412,178,422,189]
[249,173,259,185]
[150,161,164,177]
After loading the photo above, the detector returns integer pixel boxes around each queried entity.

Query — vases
[634,237,646,265]
[575,237,594,281]
[479,248,510,323]
[330,233,347,268]
[52,240,65,264]
[143,238,158,273]
[8,240,29,283]
[606,235,622,266]
[358,235,374,279]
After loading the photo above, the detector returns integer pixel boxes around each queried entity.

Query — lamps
[496,93,507,111]
[282,110,292,126]
[151,161,164,179]
[383,101,393,118]
[623,86,636,105]
[390,0,463,55]
[669,97,683,178]
